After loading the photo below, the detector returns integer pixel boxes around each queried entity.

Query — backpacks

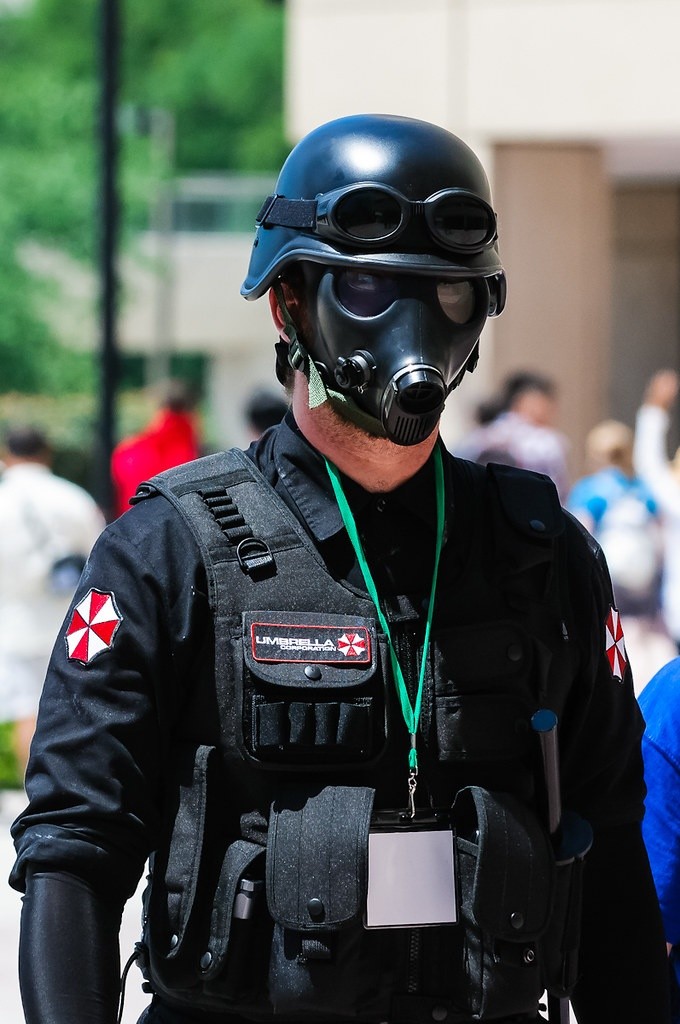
[592,472,663,599]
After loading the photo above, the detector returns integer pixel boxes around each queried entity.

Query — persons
[452,364,680,704]
[108,345,207,520]
[0,113,680,1024]
[636,656,680,1024]
[244,387,289,449]
[0,416,108,787]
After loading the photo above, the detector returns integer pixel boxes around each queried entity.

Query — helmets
[239,113,507,318]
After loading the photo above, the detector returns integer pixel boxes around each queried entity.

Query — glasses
[305,180,497,257]
